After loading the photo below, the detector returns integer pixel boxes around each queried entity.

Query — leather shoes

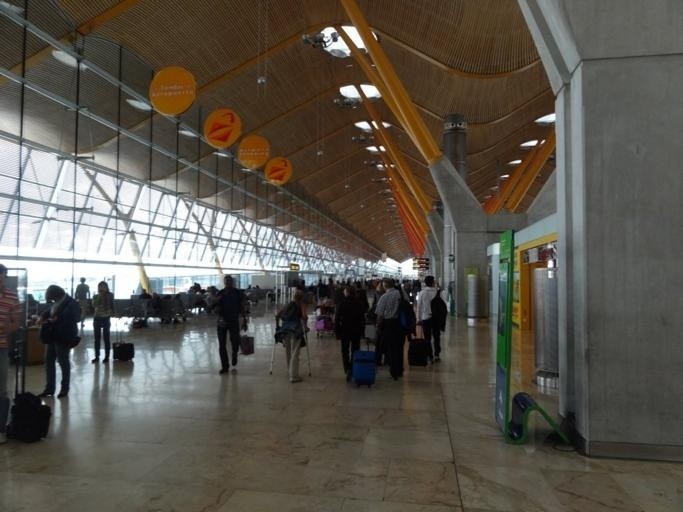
[220,368,228,374]
[232,354,237,366]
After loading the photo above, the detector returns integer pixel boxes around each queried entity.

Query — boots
[58,371,70,397]
[39,369,55,396]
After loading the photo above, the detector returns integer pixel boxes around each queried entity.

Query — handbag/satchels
[315,319,333,330]
[240,335,253,354]
[399,298,416,336]
[431,297,447,331]
[368,304,377,321]
[40,321,63,343]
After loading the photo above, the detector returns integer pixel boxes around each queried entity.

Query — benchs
[86,286,270,324]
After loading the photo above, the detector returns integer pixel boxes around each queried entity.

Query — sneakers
[103,358,110,362]
[431,357,440,364]
[290,377,302,382]
[92,358,99,362]
[0,433,7,444]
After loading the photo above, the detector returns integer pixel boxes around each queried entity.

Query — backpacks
[281,302,297,321]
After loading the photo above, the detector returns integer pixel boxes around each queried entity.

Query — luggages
[113,300,134,362]
[352,337,375,388]
[7,340,50,442]
[408,323,428,366]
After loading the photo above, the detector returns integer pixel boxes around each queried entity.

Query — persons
[92,281,116,363]
[0,264,23,443]
[75,278,90,327]
[34,284,78,398]
